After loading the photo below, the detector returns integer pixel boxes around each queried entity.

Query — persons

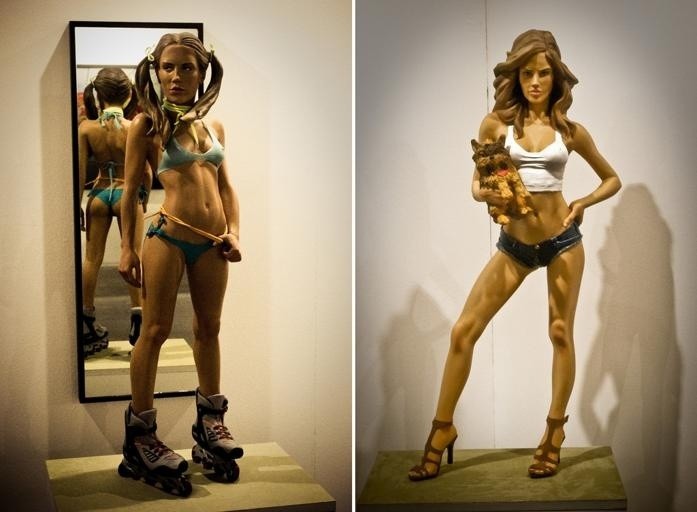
[77,66,153,347]
[407,28,624,480]
[118,32,244,476]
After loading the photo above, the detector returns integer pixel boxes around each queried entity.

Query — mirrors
[68,21,205,404]
